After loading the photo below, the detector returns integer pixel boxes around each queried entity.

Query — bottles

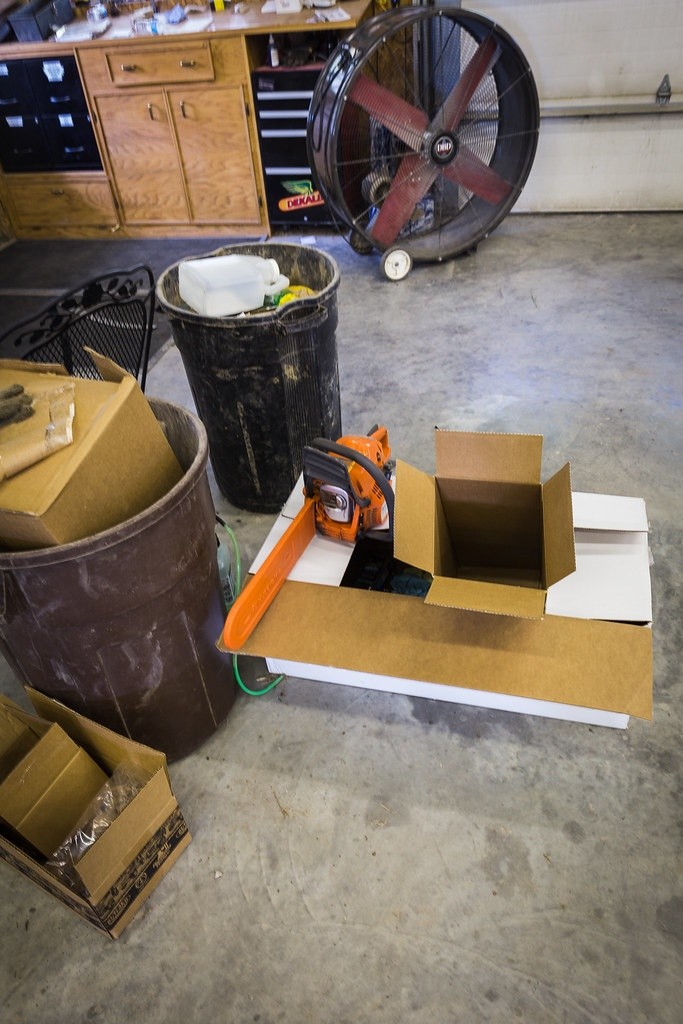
[268,33,280,67]
[175,253,291,317]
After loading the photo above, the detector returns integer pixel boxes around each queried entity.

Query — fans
[305,5,540,282]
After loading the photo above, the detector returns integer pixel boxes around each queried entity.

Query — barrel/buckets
[0,399,238,768]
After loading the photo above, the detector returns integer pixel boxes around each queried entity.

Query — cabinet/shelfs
[0,0,413,238]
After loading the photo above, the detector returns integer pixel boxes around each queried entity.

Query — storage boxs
[393,427,576,620]
[0,345,184,551]
[213,468,653,729]
[0,694,113,861]
[0,686,193,940]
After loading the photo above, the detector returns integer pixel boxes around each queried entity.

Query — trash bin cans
[155,242,344,515]
[1,395,239,765]
